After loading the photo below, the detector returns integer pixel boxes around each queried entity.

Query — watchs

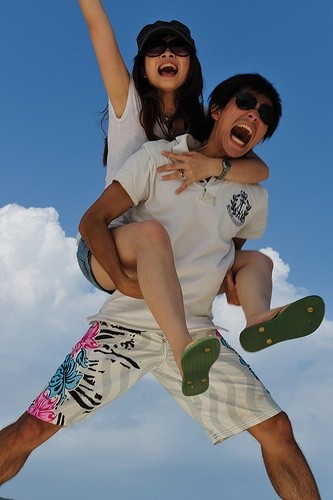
[216,158,232,179]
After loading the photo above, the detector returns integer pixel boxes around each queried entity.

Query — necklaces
[160,112,182,127]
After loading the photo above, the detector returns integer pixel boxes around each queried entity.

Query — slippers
[180,337,221,396]
[239,295,326,353]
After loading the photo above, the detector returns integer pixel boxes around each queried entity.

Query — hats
[136,20,191,52]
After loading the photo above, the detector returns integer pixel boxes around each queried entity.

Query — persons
[0,71,321,500]
[76,1,325,397]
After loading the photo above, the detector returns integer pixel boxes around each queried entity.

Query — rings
[181,170,185,179]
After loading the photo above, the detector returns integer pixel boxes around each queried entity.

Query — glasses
[234,91,276,126]
[143,39,191,58]
